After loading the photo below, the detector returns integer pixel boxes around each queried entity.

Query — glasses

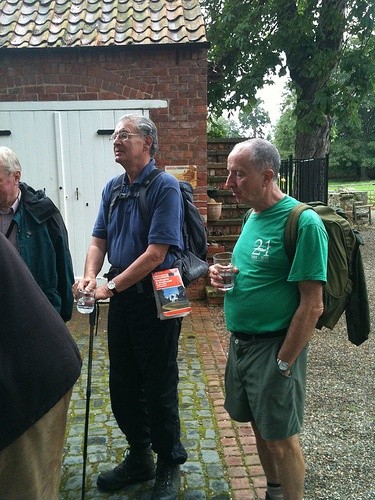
[108,131,139,142]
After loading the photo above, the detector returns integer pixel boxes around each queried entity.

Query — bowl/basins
[207,203,222,221]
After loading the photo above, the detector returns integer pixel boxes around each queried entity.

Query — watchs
[108,279,119,295]
[276,359,291,371]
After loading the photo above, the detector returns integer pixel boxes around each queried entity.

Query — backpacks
[107,167,209,291]
[244,200,358,329]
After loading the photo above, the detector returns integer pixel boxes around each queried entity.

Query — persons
[0,146,75,321]
[73,113,188,500]
[0,230,82,500]
[208,138,329,500]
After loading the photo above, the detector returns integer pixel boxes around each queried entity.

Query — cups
[213,252,235,290]
[77,288,95,314]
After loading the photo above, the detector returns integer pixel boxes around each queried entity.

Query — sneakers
[151,462,180,500]
[97,448,156,491]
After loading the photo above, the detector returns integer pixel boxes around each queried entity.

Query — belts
[233,330,278,343]
